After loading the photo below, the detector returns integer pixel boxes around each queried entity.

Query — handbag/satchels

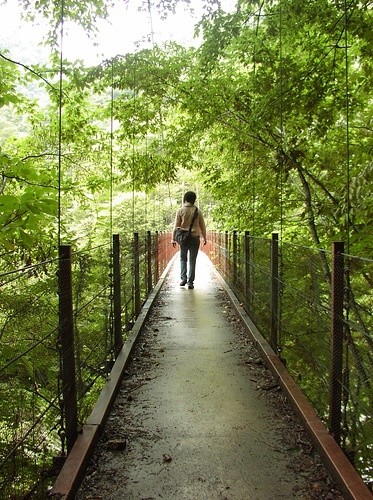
[175,228,190,247]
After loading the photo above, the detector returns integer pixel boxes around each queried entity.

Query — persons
[171,191,207,289]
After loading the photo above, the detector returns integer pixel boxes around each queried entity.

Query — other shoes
[180,280,187,286]
[188,282,194,289]
